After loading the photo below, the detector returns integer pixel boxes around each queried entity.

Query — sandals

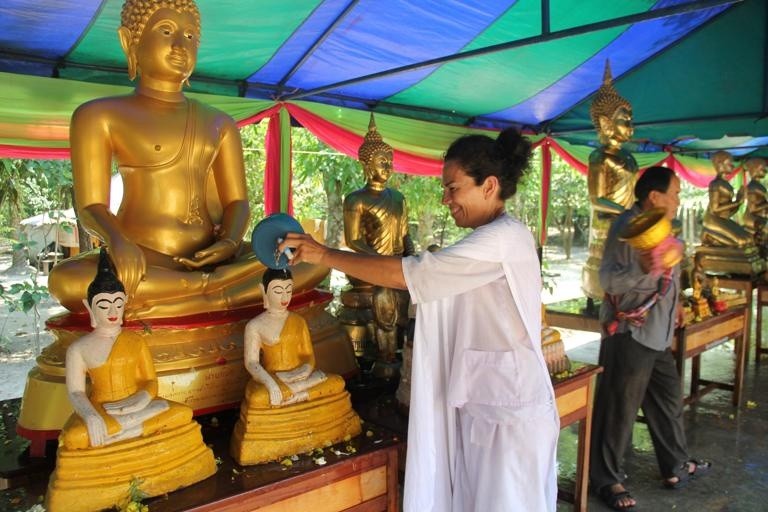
[600,484,640,512]
[662,457,713,490]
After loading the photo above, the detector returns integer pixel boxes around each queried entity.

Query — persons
[343,112,415,308]
[588,58,640,260]
[690,252,713,297]
[244,267,345,409]
[587,166,714,512]
[61,246,194,451]
[48,0,332,320]
[279,126,561,512]
[737,158,768,246]
[372,288,399,364]
[700,151,755,248]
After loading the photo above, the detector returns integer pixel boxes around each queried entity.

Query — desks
[0,358,604,512]
[544,287,751,424]
